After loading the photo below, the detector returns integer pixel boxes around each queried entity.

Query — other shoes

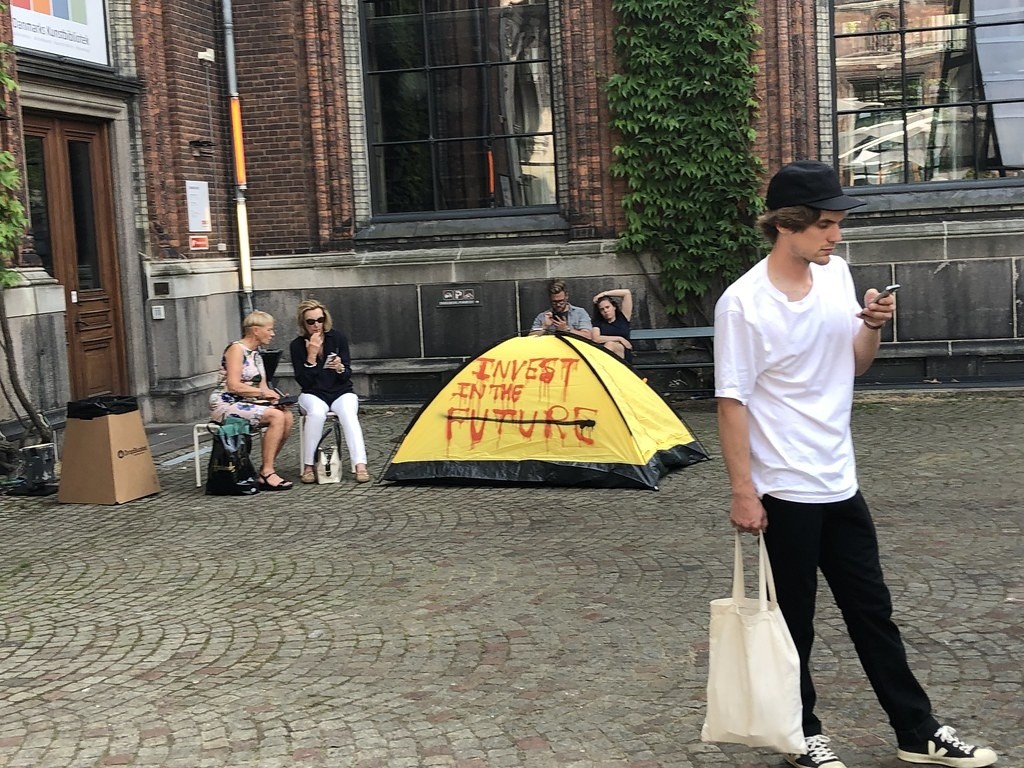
[355,465,369,483]
[302,467,315,483]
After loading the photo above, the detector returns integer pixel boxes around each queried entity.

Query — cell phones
[553,312,562,324]
[856,284,901,319]
[323,354,337,369]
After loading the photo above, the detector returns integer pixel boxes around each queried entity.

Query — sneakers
[897,722,998,768]
[783,734,849,768]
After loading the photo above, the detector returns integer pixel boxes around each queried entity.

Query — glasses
[304,316,325,325]
[550,296,566,305]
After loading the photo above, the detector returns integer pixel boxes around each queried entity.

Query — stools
[193,423,267,489]
[299,412,341,478]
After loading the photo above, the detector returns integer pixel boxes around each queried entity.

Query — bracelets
[336,364,345,374]
[258,388,264,399]
[864,320,882,330]
[567,328,571,332]
[304,363,317,368]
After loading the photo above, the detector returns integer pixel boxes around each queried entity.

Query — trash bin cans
[56,395,164,505]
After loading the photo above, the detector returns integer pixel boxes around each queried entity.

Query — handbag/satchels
[313,423,343,485]
[206,419,258,496]
[699,530,809,756]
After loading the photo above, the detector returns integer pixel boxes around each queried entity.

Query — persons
[592,289,633,362]
[208,311,294,489]
[713,159,997,768]
[528,282,592,340]
[290,299,370,482]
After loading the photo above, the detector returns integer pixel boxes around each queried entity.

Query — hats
[767,160,867,211]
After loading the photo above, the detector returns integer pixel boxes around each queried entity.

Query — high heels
[258,472,292,490]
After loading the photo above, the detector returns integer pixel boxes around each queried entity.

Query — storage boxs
[57,395,162,505]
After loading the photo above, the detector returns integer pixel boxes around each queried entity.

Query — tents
[374,330,713,492]
